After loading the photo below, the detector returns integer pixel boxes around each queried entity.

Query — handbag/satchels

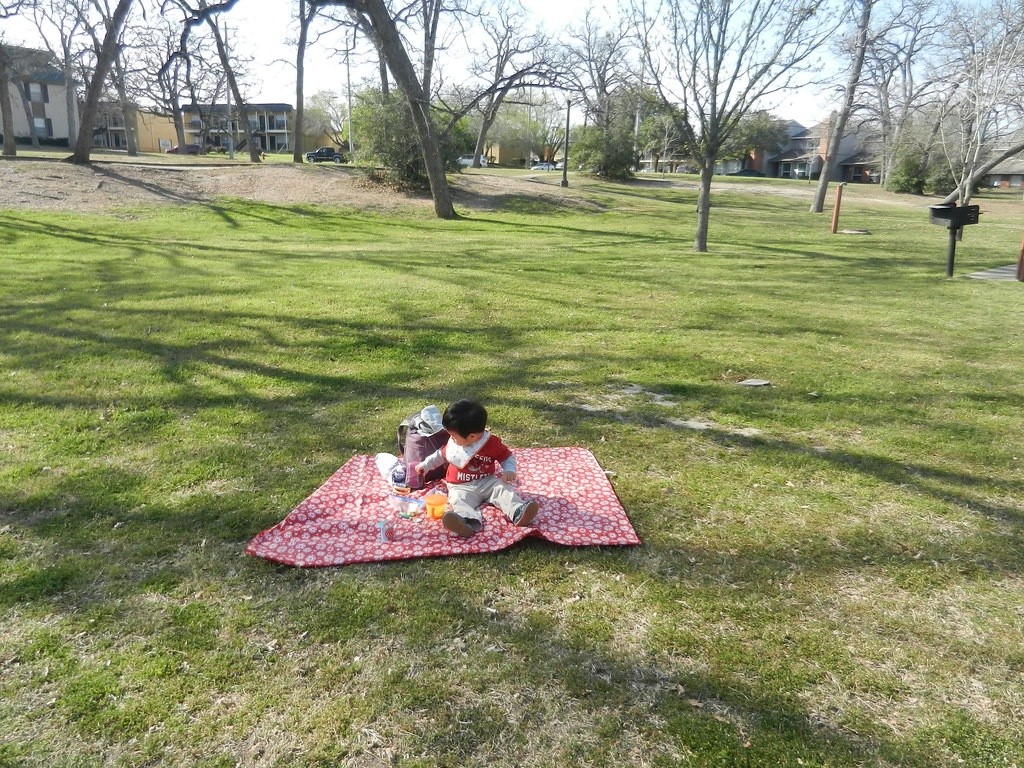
[398,405,452,482]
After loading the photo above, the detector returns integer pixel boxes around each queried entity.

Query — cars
[530,162,555,170]
[555,162,564,170]
[165,143,202,155]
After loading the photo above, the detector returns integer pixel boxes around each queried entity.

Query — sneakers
[513,498,538,527]
[442,510,473,537]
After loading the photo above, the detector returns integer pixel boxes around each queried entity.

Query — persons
[415,399,539,539]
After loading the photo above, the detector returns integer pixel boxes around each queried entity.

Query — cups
[426,494,447,519]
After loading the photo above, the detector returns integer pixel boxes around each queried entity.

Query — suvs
[306,146,346,163]
[447,153,488,168]
